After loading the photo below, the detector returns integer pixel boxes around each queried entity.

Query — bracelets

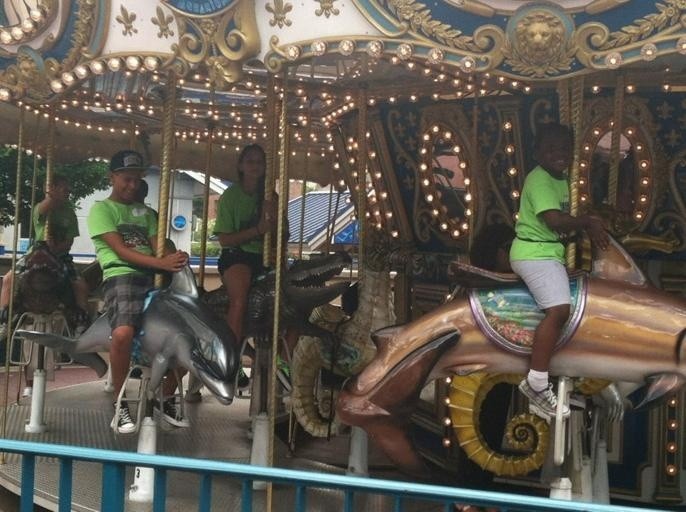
[256,222,261,235]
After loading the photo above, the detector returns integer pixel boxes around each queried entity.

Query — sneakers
[0,322,8,342]
[23,387,32,398]
[113,398,137,434]
[550,379,587,412]
[276,368,293,393]
[517,377,571,419]
[237,362,250,391]
[153,395,190,428]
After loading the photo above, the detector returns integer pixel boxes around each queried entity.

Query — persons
[132,178,159,223]
[1,175,91,321]
[509,122,610,419]
[210,144,313,392]
[86,149,191,433]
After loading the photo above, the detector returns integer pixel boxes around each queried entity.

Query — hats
[110,150,150,174]
